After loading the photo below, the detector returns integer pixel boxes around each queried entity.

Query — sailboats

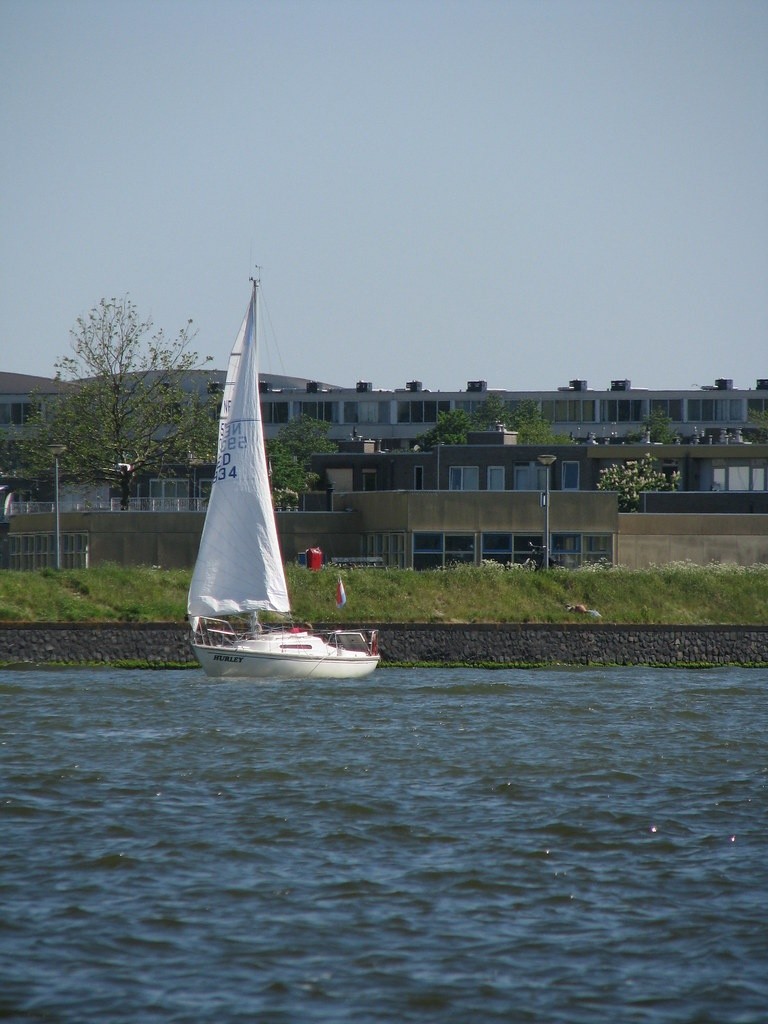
[183,266,381,678]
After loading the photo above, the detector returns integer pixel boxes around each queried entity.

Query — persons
[290,620,313,633]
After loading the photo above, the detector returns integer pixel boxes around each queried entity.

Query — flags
[336,579,346,608]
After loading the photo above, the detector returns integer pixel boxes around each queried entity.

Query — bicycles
[523,540,565,571]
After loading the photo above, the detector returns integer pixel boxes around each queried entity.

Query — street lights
[538,454,558,575]
[48,445,67,570]
